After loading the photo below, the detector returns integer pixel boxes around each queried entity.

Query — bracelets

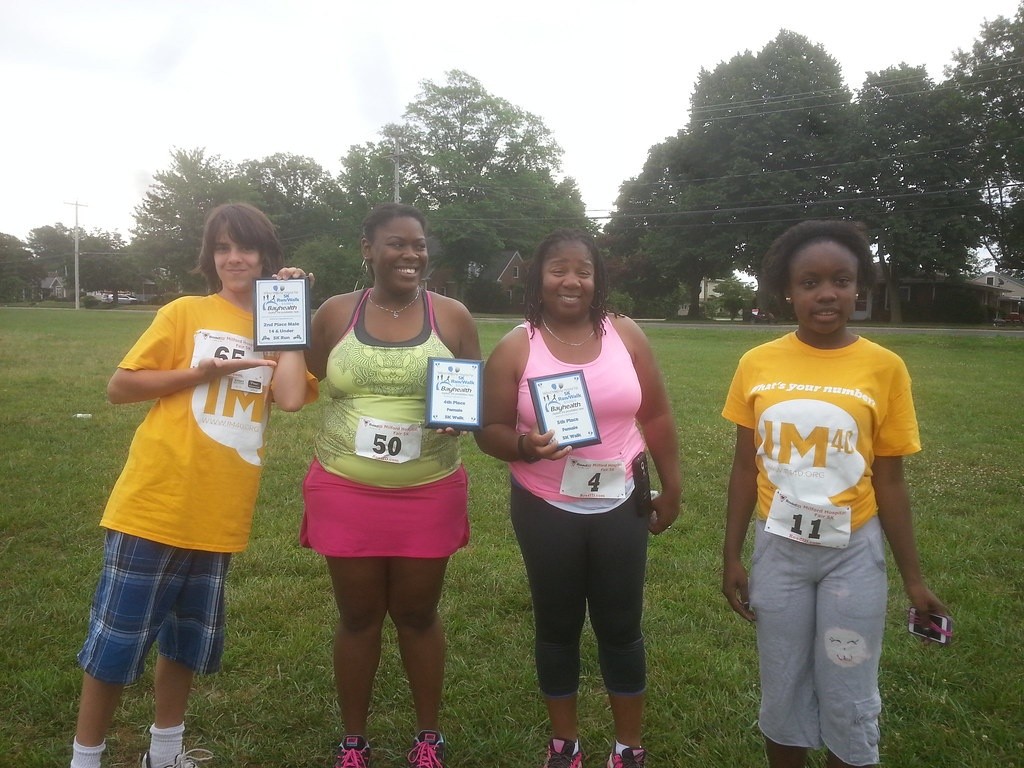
[518,433,541,464]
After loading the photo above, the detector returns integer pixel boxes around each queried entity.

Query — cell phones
[632,451,654,516]
[909,607,953,643]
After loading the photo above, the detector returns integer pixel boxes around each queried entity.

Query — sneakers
[142,745,213,768]
[607,739,646,768]
[543,738,582,768]
[407,730,445,768]
[334,735,371,768]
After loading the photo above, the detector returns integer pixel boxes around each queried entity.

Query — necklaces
[542,319,594,346]
[368,287,420,319]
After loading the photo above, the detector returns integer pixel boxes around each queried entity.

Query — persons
[473,227,680,768]
[69,202,319,768]
[300,203,482,768]
[720,220,952,768]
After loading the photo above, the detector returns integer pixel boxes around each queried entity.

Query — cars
[751,308,775,324]
[108,293,139,304]
